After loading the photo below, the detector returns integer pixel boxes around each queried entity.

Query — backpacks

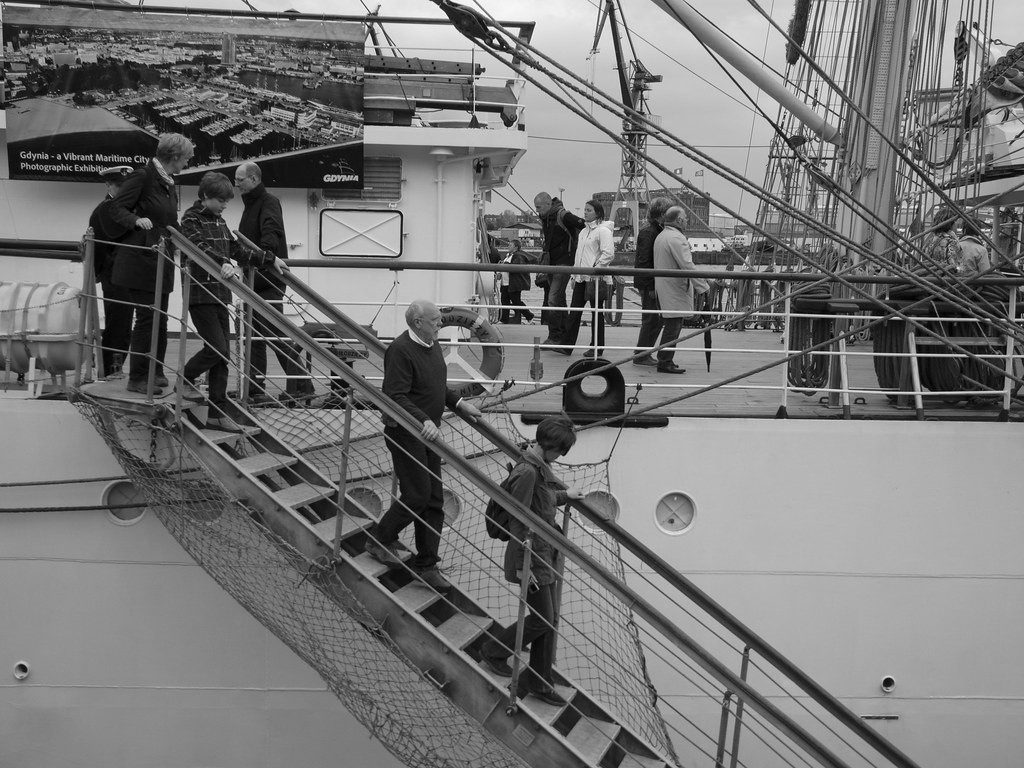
[485,462,542,542]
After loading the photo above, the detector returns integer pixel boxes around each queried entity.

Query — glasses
[234,174,254,183]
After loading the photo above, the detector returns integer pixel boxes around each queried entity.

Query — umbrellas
[700,291,712,373]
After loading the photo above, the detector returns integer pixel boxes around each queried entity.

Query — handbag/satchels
[99,201,134,240]
[585,280,609,301]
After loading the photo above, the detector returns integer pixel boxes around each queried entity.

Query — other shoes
[584,348,603,357]
[657,359,686,374]
[539,338,562,350]
[480,645,513,677]
[633,351,657,367]
[127,373,169,395]
[531,684,567,706]
[227,389,266,399]
[552,344,572,355]
[278,391,315,402]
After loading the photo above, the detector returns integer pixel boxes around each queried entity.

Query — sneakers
[364,539,406,568]
[173,380,205,402]
[412,564,452,592]
[206,416,246,433]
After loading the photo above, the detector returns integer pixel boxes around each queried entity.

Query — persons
[86,134,317,432]
[478,418,585,707]
[364,299,482,591]
[482,191,710,373]
[922,209,991,272]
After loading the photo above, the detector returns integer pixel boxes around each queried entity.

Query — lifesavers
[432,306,505,399]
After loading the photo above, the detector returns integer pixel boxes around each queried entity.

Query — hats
[99,165,134,179]
